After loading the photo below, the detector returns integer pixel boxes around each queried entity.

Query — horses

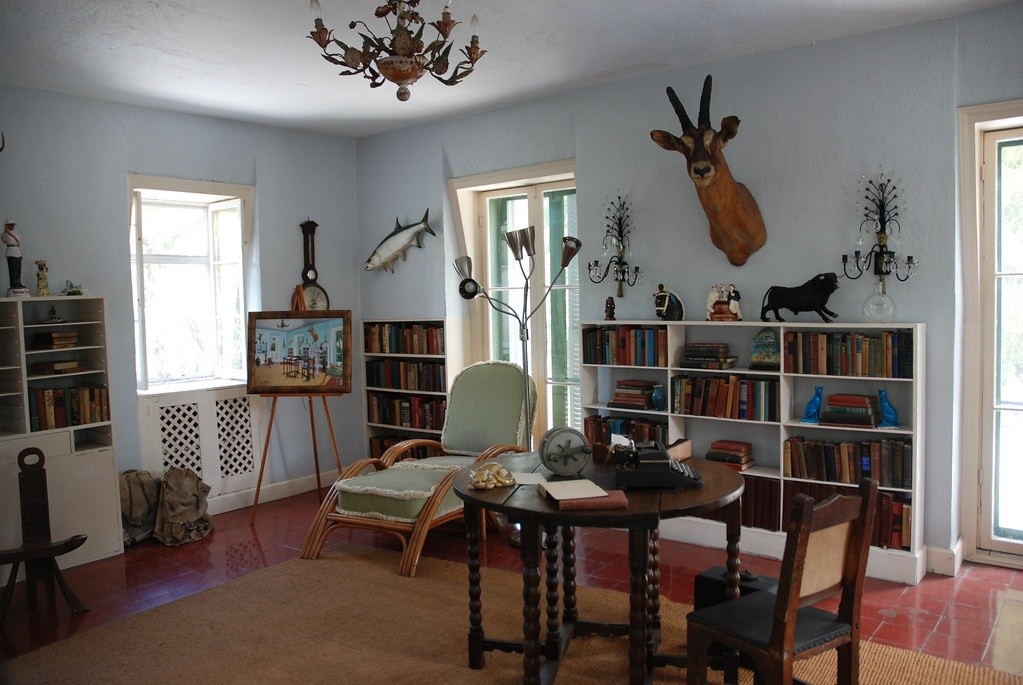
[652,284,683,321]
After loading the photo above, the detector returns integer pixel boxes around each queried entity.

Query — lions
[760,272,841,324]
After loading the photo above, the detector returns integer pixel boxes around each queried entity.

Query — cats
[468,461,516,491]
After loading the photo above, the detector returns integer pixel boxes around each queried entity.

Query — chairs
[300,360,538,577]
[686,478,878,685]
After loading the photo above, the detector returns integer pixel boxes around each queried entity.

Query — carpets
[0,544,1023,685]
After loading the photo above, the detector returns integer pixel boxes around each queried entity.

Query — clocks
[293,220,329,311]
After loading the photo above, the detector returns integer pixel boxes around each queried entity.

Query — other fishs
[364,207,437,274]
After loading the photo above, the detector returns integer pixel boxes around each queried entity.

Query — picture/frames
[246,309,352,395]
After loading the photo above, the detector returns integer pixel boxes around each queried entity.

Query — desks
[453,448,745,685]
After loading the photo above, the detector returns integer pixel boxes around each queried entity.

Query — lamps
[840,174,920,294]
[586,195,643,297]
[307,0,488,101]
[451,227,583,451]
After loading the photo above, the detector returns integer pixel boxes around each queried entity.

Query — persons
[705,283,743,321]
[2,217,26,289]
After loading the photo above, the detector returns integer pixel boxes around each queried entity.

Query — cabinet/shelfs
[576,321,927,587]
[359,319,447,463]
[0,293,123,586]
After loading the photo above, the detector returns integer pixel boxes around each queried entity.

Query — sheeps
[652,74,768,265]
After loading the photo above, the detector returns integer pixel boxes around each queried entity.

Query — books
[784,436,912,490]
[35,331,77,349]
[584,415,669,449]
[28,386,108,432]
[367,392,447,432]
[538,479,629,510]
[783,481,912,550]
[783,329,913,379]
[366,359,446,393]
[706,440,755,472]
[606,380,657,409]
[670,374,780,422]
[703,476,780,533]
[818,394,879,429]
[34,360,85,375]
[365,323,445,355]
[679,343,738,369]
[369,435,442,463]
[582,325,668,367]
